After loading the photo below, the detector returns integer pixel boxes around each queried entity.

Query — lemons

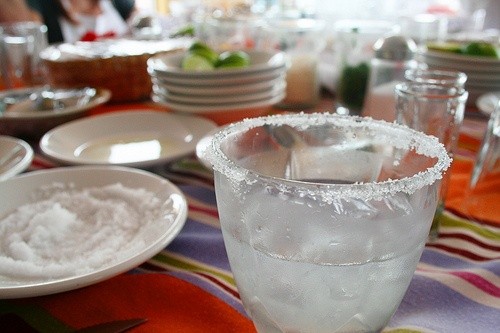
[181,41,252,72]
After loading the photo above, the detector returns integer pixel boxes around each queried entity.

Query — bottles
[362,31,419,128]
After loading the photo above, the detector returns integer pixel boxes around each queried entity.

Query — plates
[0,165,189,300]
[0,135,34,182]
[0,84,111,125]
[146,46,288,114]
[39,110,218,168]
[427,48,500,104]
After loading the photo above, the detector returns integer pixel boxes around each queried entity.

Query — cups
[0,19,48,89]
[394,81,469,153]
[192,12,402,116]
[202,112,454,333]
[403,69,468,139]
[466,106,500,229]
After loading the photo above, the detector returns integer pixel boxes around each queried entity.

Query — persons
[0,0,142,47]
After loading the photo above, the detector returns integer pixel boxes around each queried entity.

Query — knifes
[75,318,147,333]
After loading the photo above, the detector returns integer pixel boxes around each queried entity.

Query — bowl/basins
[40,41,193,103]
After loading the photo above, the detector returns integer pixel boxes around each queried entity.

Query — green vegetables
[426,40,500,59]
[334,60,370,115]
